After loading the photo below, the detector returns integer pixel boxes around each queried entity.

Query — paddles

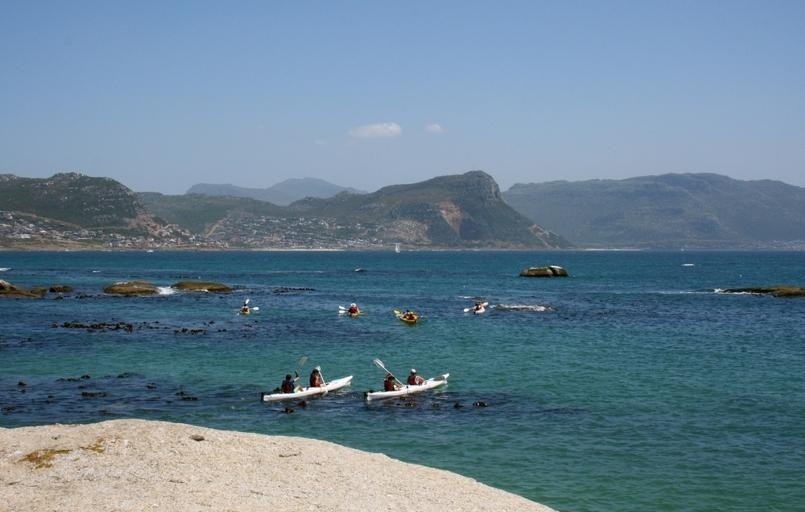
[338,306,348,314]
[374,359,406,387]
[464,302,488,312]
[316,366,326,386]
[250,307,260,311]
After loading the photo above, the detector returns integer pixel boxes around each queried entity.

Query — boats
[393,310,417,324]
[349,306,360,317]
[261,374,354,403]
[363,373,450,401]
[240,303,250,315]
[472,302,485,315]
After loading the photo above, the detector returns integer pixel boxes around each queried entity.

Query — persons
[383,373,402,392]
[280,373,308,394]
[240,304,250,313]
[310,369,323,387]
[348,302,361,314]
[403,309,410,318]
[474,301,482,311]
[407,367,425,386]
[405,311,419,320]
